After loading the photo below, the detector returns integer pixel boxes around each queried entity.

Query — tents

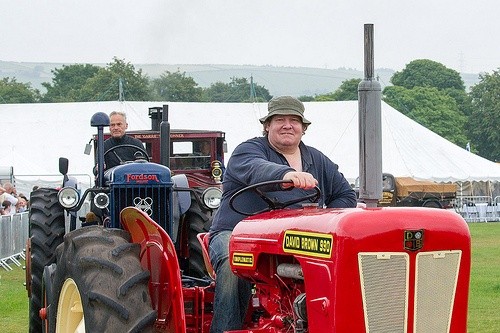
[0,100,500,208]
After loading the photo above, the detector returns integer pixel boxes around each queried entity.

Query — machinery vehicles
[22,103,228,333]
[40,23,472,333]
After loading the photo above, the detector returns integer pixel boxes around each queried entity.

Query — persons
[208,96,357,333]
[86,112,148,224]
[487,198,497,213]
[0,182,39,215]
[468,198,479,214]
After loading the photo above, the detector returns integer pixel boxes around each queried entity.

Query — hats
[259,95,312,125]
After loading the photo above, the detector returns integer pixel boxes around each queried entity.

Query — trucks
[350,172,457,210]
